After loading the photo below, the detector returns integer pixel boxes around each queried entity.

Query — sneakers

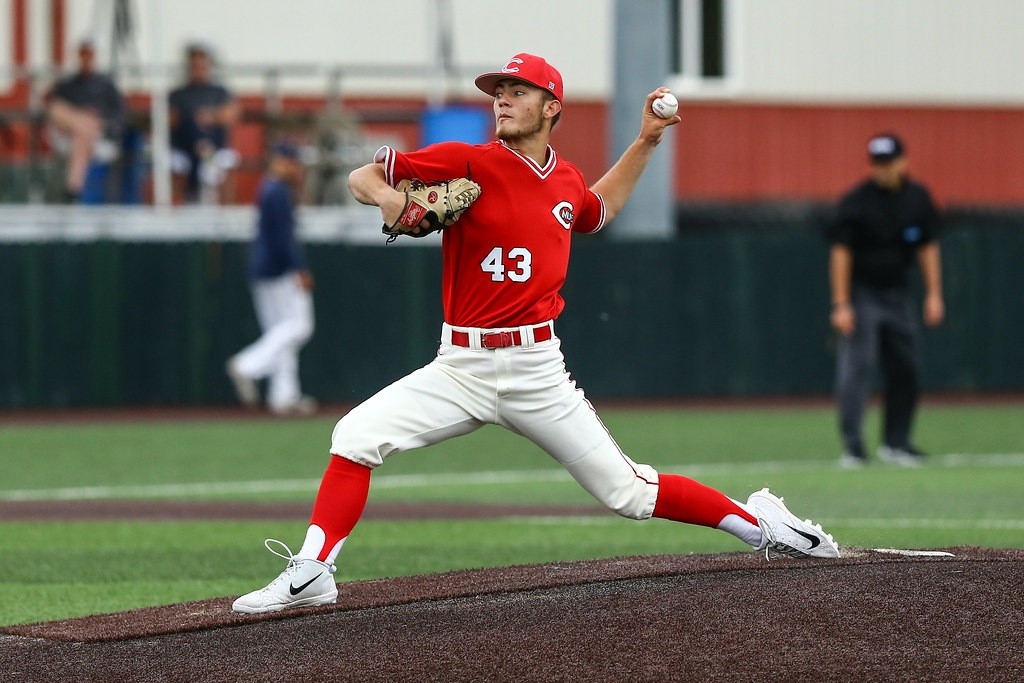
[231,537,339,615]
[745,485,841,558]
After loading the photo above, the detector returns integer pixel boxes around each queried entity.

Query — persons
[39,41,127,205]
[166,48,241,204]
[828,133,948,468]
[230,51,840,614]
[224,140,320,417]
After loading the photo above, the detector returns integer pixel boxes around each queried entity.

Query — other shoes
[842,451,865,472]
[225,359,259,407]
[286,397,316,413]
[879,442,930,470]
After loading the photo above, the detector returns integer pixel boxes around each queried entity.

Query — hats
[867,132,904,165]
[474,53,562,108]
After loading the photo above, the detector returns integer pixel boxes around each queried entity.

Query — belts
[450,325,552,350]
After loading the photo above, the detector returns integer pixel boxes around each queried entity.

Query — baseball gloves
[382,177,482,238]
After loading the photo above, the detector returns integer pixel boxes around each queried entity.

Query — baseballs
[652,93,679,119]
